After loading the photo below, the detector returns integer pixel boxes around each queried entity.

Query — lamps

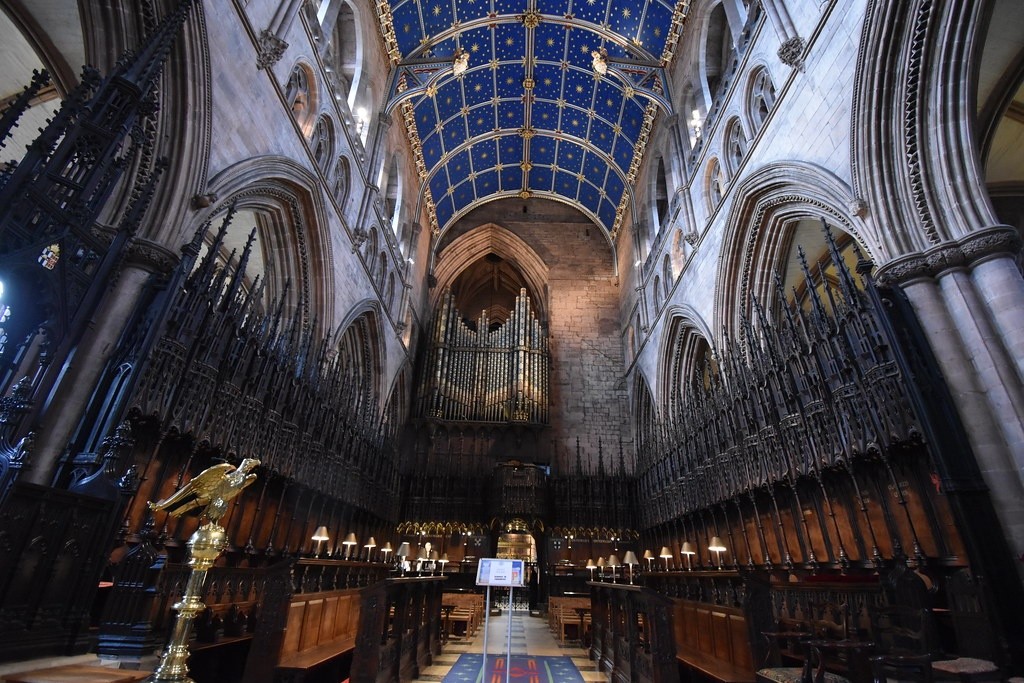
[311,526,449,578]
[586,537,727,585]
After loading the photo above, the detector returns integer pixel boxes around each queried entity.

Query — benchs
[548,597,591,645]
[442,592,484,641]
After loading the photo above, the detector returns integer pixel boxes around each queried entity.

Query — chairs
[91,543,440,683]
[592,571,933,683]
